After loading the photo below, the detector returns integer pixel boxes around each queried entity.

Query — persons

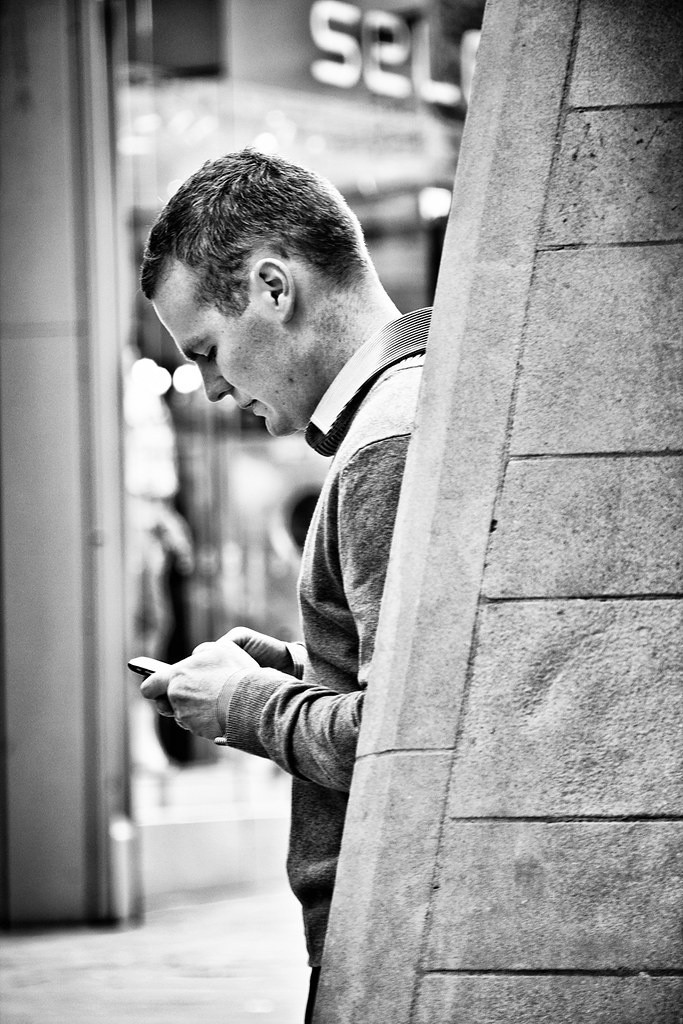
[138,149,432,1024]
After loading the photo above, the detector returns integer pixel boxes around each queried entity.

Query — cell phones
[128,657,170,679]
